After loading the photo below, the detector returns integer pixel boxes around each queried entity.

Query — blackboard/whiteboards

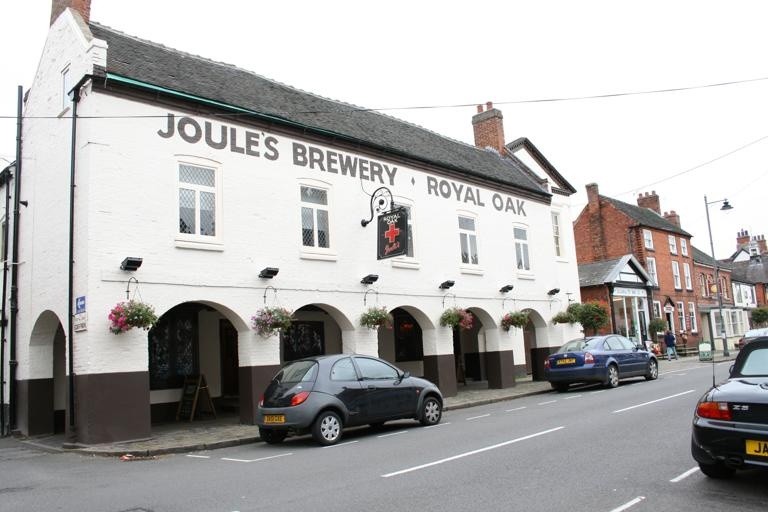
[177,375,202,414]
[197,374,216,412]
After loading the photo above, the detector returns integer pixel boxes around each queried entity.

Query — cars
[691,328,768,479]
[256,352,444,446]
[544,333,658,393]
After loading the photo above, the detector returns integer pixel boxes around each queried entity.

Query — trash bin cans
[699,342,712,361]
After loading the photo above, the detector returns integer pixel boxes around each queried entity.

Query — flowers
[252,309,293,341]
[500,309,532,330]
[440,307,472,331]
[109,301,159,336]
[359,307,395,330]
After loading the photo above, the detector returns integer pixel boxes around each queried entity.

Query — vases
[130,320,143,327]
[272,322,282,329]
[374,319,384,326]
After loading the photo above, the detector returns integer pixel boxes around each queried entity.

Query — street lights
[704,195,734,357]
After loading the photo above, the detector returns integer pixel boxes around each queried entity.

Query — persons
[664,330,678,361]
